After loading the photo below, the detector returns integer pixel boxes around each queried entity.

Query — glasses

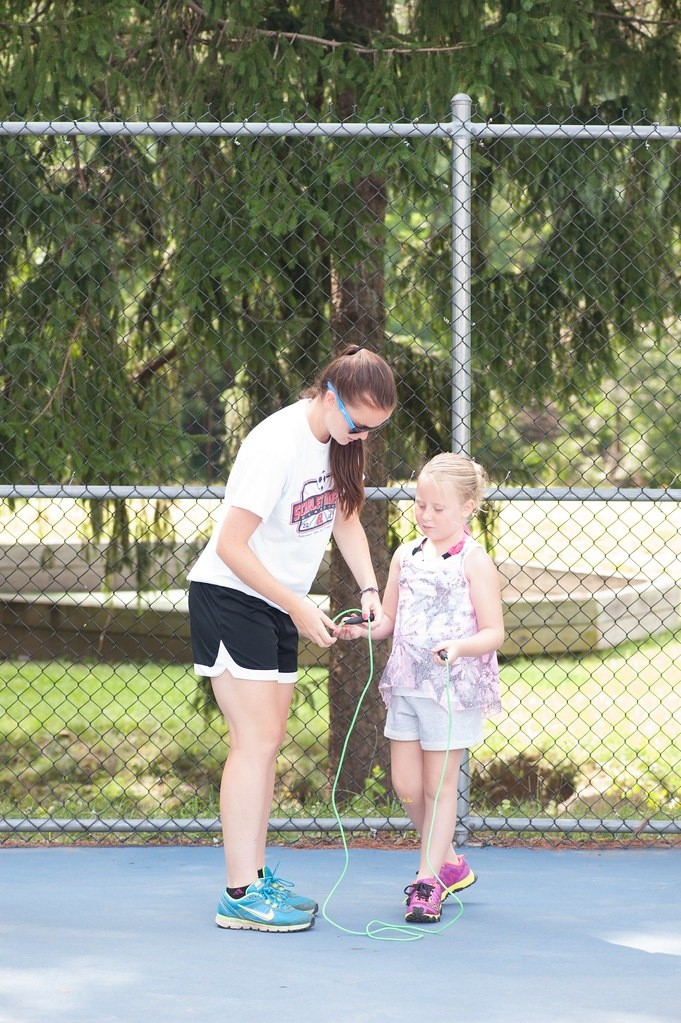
[326,380,390,436]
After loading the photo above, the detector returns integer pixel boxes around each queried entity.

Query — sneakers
[259,861,318,914]
[404,855,476,906]
[404,877,442,923]
[215,879,315,932]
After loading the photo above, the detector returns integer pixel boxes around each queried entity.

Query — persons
[184,344,397,932]
[332,451,505,923]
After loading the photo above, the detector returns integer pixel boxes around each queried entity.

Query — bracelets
[359,587,379,596]
